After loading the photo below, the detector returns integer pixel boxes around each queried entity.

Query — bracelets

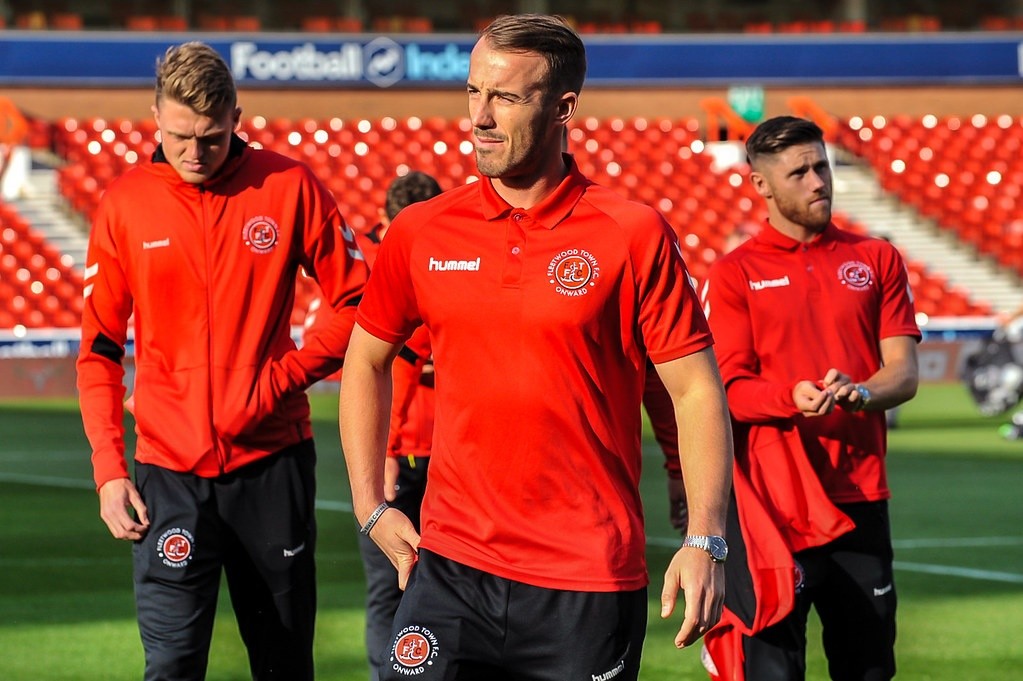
[853,383,872,412]
[359,503,388,535]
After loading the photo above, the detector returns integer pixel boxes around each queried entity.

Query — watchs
[681,535,729,564]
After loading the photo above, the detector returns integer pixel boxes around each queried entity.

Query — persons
[707,114,924,680]
[76,40,373,680]
[337,13,737,681]
